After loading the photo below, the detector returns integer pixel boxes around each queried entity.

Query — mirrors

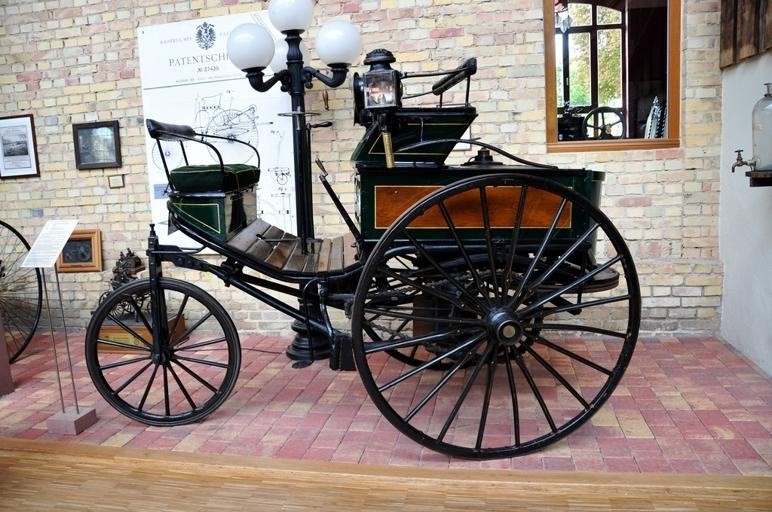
[542,0,680,151]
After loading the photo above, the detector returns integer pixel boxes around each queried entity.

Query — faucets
[732,148,756,174]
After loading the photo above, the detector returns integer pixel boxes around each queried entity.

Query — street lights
[225,0,365,359]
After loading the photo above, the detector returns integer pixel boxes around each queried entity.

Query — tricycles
[82,49,648,464]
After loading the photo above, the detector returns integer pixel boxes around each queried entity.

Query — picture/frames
[0,114,40,180]
[71,120,122,171]
[54,227,102,273]
